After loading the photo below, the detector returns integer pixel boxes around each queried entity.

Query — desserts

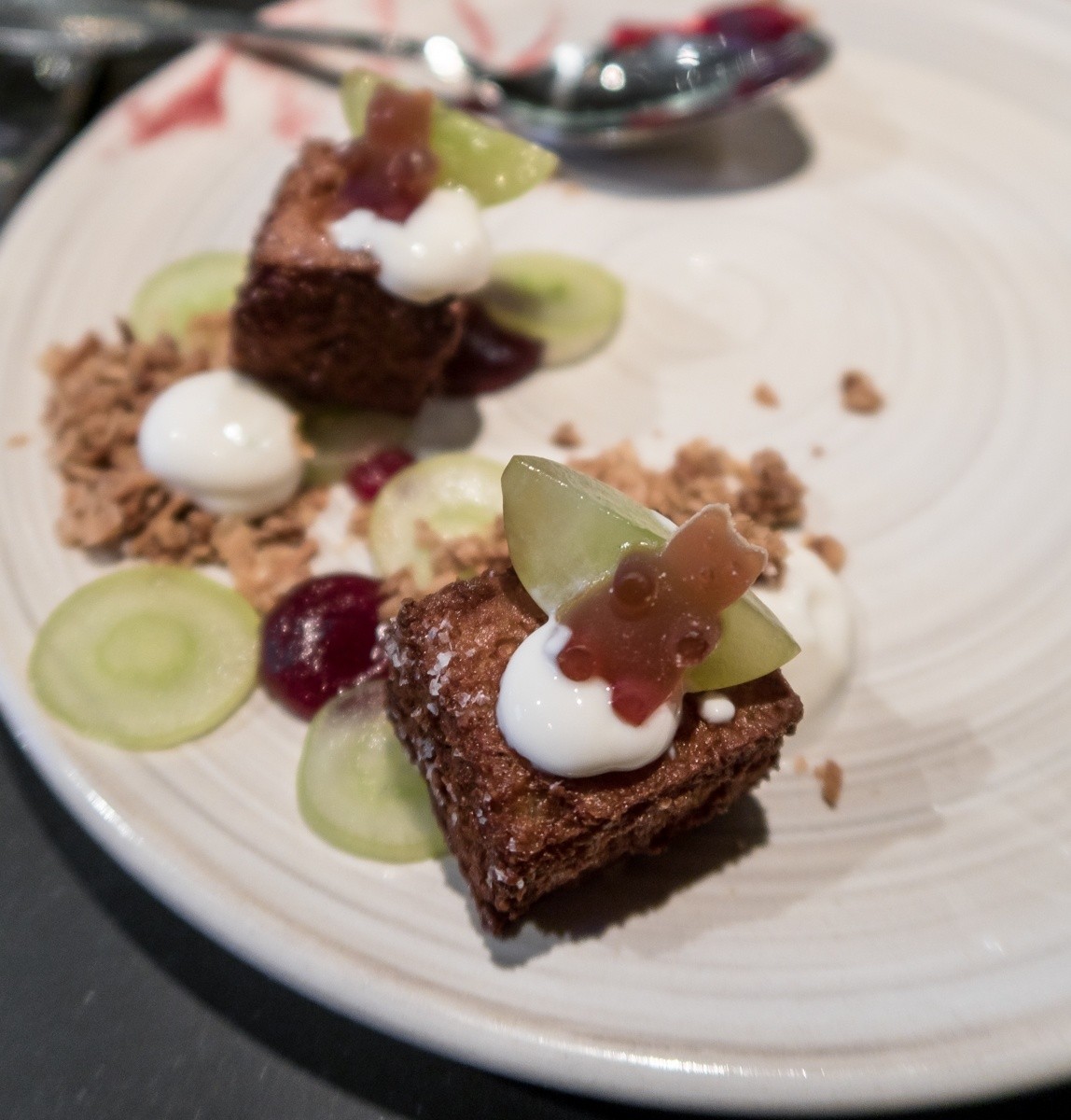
[225,68,564,413]
[376,447,809,930]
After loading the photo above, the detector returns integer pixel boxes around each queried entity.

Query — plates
[2,2,1071,1120]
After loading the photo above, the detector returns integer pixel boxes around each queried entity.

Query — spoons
[0,2,837,160]
